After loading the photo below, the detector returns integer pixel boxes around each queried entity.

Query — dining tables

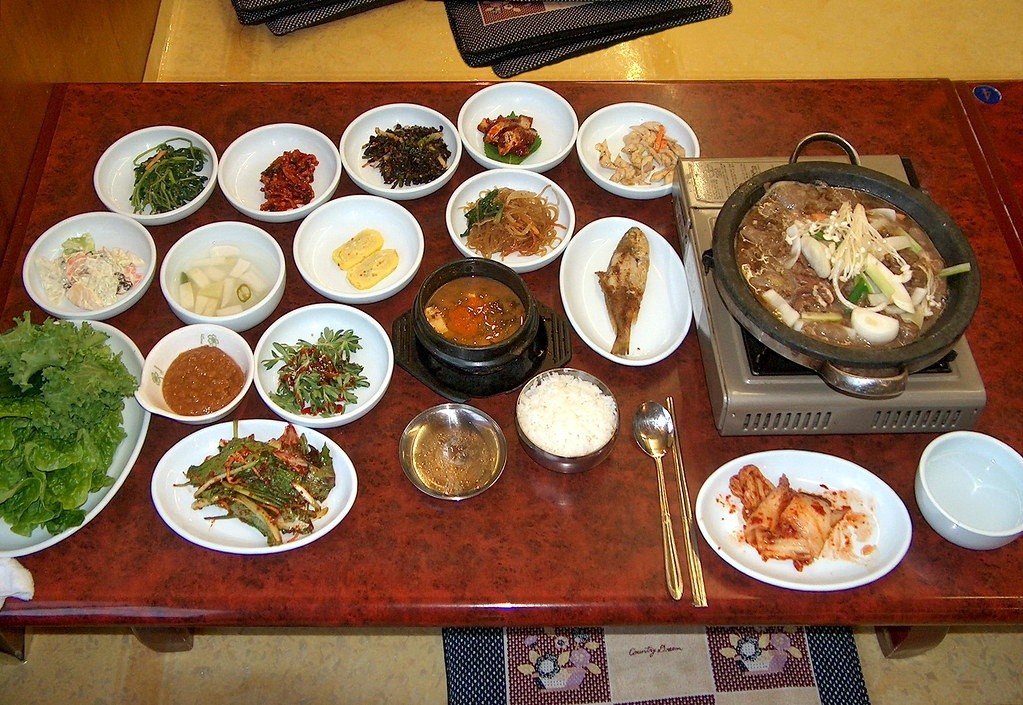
[1,78,1023,633]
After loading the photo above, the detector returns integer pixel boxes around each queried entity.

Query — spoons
[632,400,684,600]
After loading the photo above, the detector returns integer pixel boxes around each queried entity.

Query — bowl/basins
[134,323,254,425]
[914,431,1022,550]
[160,221,287,333]
[410,257,539,375]
[515,368,620,474]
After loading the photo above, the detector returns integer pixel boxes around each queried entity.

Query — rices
[518,372,618,456]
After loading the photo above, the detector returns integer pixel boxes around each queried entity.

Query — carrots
[653,125,668,152]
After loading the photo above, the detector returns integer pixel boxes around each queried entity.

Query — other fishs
[595,226,650,356]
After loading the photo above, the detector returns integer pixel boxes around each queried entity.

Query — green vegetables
[129,136,209,216]
[0,310,141,536]
[171,420,336,546]
[484,110,542,165]
[260,326,370,418]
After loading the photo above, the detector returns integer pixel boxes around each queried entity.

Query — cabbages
[726,464,849,569]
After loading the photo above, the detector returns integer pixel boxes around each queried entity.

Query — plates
[1,319,151,558]
[695,449,913,592]
[457,82,579,174]
[218,123,342,223]
[293,195,424,305]
[398,403,508,500]
[22,211,157,321]
[339,103,462,201]
[445,168,575,274]
[94,126,218,226]
[151,419,358,554]
[559,217,693,366]
[254,303,394,428]
[576,102,701,200]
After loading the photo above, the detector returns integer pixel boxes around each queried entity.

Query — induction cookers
[671,154,987,437]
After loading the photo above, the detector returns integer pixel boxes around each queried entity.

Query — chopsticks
[666,396,708,607]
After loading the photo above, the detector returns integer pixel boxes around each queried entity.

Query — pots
[713,132,981,396]
